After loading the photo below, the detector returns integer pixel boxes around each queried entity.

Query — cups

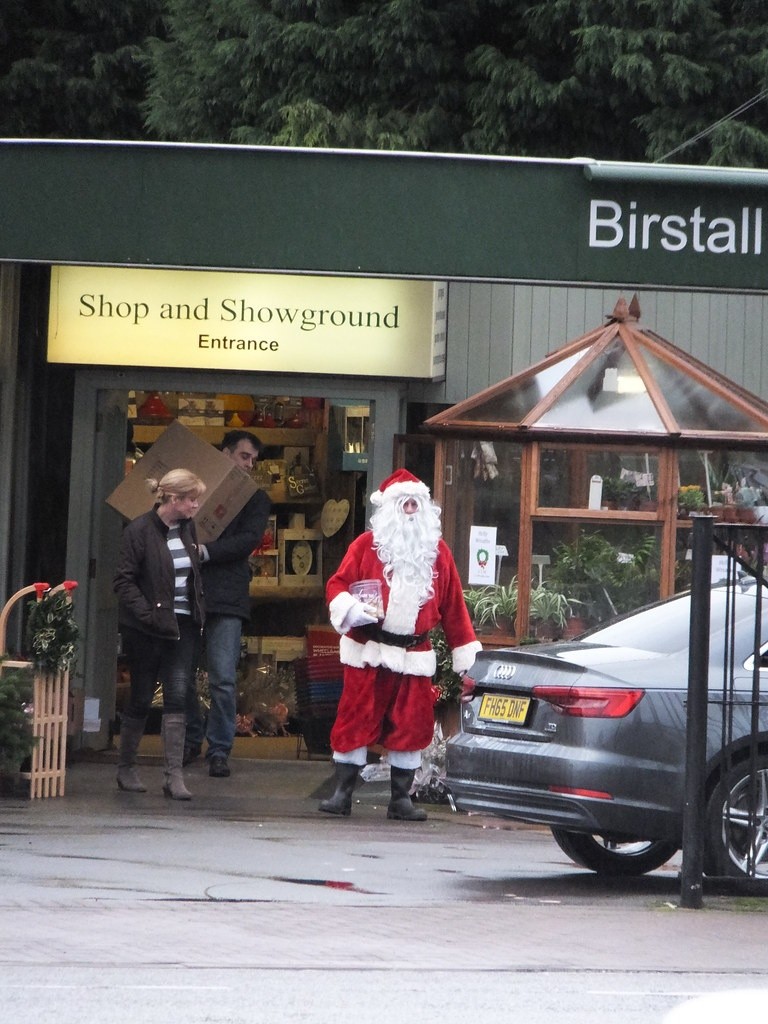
[349,579,386,621]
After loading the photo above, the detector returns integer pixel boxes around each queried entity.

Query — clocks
[285,540,319,576]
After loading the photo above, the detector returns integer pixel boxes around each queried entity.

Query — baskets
[293,652,347,755]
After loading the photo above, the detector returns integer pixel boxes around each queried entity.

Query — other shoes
[182,745,196,766]
[208,757,231,776]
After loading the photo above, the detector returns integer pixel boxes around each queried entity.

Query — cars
[443,572,767,891]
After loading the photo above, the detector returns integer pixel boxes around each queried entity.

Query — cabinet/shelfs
[0,581,77,801]
[134,423,327,661]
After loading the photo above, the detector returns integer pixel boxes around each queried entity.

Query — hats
[370,469,429,506]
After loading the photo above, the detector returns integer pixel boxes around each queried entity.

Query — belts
[360,623,429,648]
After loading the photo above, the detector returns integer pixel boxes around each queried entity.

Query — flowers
[680,483,704,505]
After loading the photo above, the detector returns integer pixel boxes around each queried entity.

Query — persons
[318,468,482,822]
[112,467,208,800]
[182,429,272,777]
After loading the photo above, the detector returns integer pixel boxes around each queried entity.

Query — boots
[161,713,195,798]
[117,711,148,792]
[387,749,428,822]
[319,746,367,815]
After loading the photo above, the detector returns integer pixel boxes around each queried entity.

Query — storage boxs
[278,529,324,588]
[105,420,258,546]
[255,514,277,550]
[249,549,279,586]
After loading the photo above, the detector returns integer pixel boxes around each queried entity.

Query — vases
[693,507,708,516]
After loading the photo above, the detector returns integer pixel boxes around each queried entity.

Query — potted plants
[709,466,756,522]
[603,473,657,512]
[471,576,586,642]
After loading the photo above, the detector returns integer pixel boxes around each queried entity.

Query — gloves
[346,601,379,628]
